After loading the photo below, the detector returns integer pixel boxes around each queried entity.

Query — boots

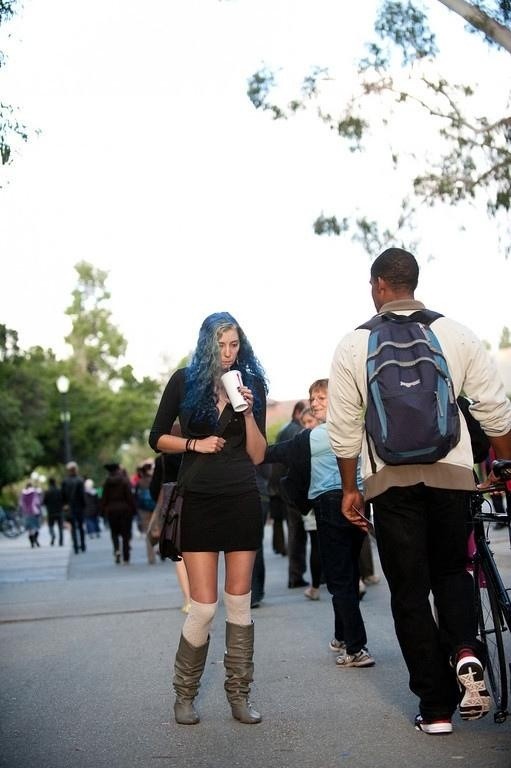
[172,632,211,726]
[224,620,265,724]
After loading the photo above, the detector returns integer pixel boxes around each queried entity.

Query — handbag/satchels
[147,481,184,561]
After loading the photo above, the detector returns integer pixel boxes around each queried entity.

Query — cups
[220,370,249,413]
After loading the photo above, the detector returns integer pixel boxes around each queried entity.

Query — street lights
[55,372,72,463]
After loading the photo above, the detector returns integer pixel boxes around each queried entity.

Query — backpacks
[356,309,463,465]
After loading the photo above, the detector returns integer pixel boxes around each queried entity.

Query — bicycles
[472,481,510,722]
[0,504,25,538]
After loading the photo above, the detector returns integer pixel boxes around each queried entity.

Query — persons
[327,250,511,734]
[62,461,87,552]
[147,310,268,725]
[250,380,380,610]
[85,477,100,539]
[19,483,41,549]
[258,379,367,601]
[104,424,184,565]
[486,447,509,531]
[43,478,65,545]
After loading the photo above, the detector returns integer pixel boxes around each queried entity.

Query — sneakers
[414,713,453,735]
[334,651,376,668]
[328,638,348,652]
[31,530,168,565]
[179,550,378,616]
[455,656,492,723]
